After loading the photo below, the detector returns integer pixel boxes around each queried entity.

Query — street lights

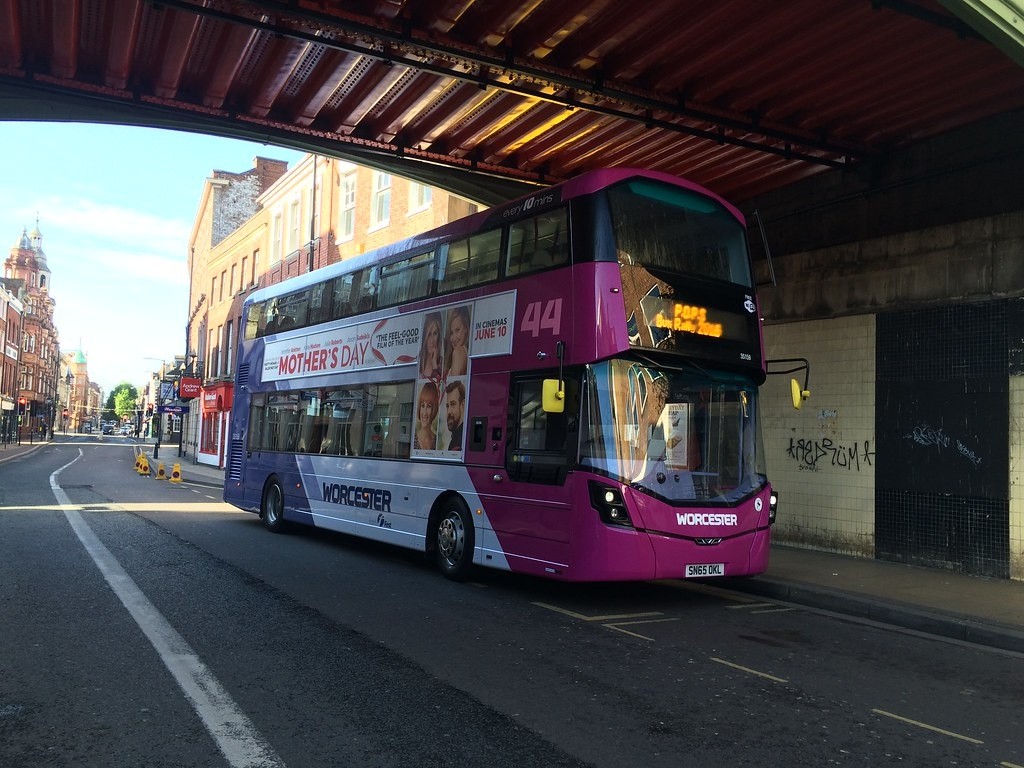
[145,356,166,381]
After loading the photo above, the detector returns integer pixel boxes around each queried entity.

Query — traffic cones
[155,463,167,480]
[133,452,151,476]
[169,463,182,481]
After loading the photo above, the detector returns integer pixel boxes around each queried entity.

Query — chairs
[256,294,370,340]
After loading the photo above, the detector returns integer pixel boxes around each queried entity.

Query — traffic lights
[17,397,26,415]
[64,409,68,418]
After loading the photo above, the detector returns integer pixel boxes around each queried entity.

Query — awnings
[157,399,189,413]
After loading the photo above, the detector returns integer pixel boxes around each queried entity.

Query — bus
[221,165,811,588]
[109,420,118,427]
[99,420,106,427]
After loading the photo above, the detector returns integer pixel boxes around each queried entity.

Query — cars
[103,425,114,436]
[119,425,135,435]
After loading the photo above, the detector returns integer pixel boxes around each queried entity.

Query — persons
[413,304,472,451]
